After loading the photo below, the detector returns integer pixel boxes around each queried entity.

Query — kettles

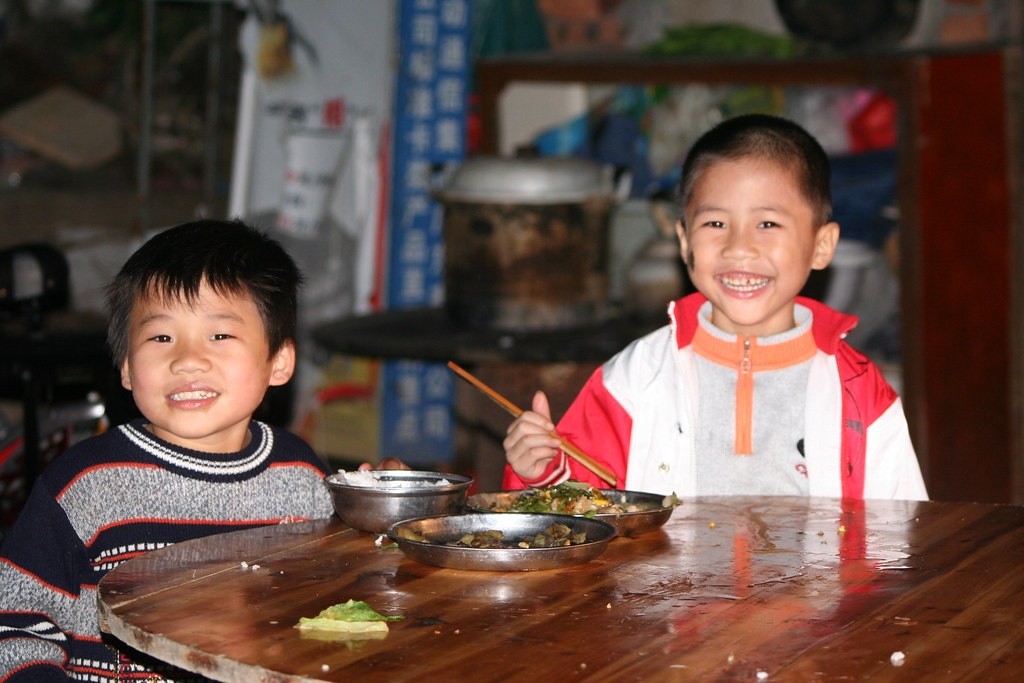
[622,199,689,317]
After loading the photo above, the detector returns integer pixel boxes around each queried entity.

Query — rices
[329,468,451,487]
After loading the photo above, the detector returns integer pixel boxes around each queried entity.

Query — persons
[0,219,413,683]
[501,113,930,501]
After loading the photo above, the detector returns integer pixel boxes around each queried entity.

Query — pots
[426,142,636,333]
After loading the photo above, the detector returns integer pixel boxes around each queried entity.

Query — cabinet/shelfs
[478,36,1023,503]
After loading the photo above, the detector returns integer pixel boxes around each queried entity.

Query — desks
[96,497,1024,683]
[315,298,670,442]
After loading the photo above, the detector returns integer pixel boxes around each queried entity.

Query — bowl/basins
[323,469,475,532]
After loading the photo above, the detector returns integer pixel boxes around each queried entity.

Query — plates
[466,488,683,538]
[386,512,618,570]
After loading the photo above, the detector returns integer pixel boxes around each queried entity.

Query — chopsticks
[448,361,617,486]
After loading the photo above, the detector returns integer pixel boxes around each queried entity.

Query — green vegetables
[292,598,406,632]
[483,484,597,520]
